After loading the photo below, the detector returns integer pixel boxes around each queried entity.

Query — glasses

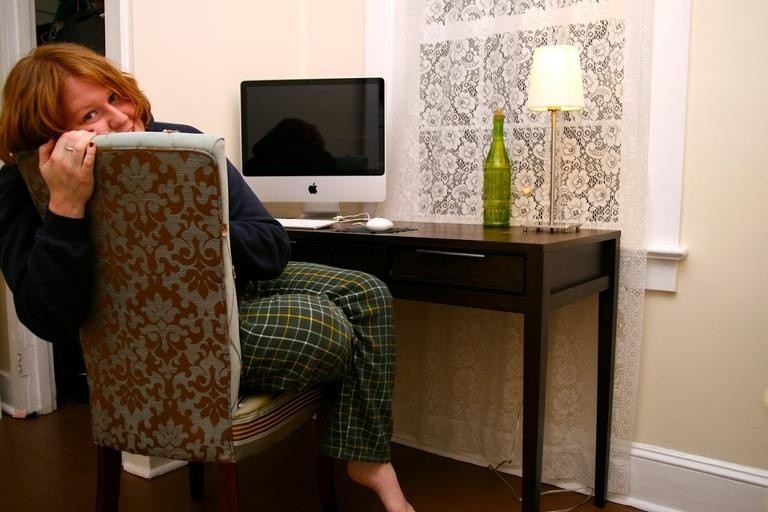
[521,43,585,235]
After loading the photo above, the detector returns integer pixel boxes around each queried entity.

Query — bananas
[275,218,338,229]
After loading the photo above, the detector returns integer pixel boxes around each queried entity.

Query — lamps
[12,131,342,510]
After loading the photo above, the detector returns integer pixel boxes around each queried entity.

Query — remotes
[366,217,394,231]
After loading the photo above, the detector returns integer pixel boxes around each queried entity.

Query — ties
[239,76,387,218]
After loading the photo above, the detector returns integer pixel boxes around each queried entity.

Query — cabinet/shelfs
[483,108,511,230]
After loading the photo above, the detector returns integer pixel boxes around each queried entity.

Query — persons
[0,42,416,512]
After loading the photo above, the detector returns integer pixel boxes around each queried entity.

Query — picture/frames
[284,210,625,509]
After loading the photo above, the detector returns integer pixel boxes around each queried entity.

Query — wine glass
[65,145,75,152]
